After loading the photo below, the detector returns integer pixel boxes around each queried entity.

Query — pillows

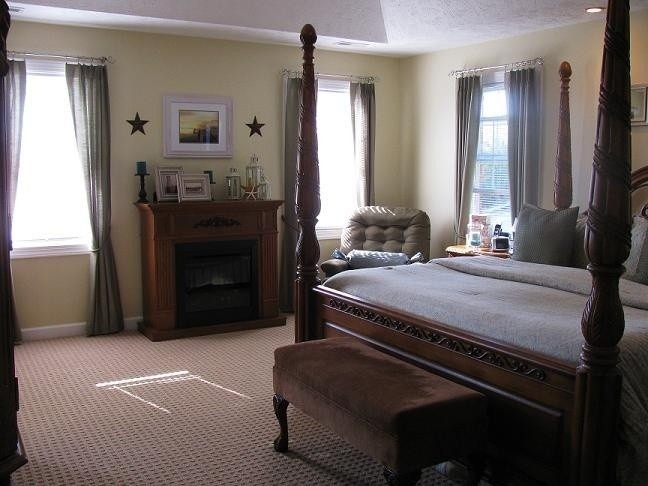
[576,213,598,268]
[509,202,580,268]
[328,243,425,270]
[623,216,648,284]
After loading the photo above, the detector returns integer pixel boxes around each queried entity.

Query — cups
[465,234,471,247]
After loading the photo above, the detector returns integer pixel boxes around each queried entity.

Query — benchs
[271,334,492,484]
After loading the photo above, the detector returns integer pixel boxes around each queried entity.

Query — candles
[135,161,147,175]
[203,169,213,182]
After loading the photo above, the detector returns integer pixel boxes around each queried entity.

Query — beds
[245,0,648,484]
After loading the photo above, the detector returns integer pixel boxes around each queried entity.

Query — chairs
[320,205,431,278]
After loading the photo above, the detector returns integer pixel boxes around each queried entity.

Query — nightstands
[443,242,514,259]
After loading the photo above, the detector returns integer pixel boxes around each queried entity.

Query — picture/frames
[161,93,235,160]
[155,165,184,203]
[176,173,211,203]
[630,82,647,127]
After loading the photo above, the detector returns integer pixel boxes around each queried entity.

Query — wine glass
[468,223,482,254]
